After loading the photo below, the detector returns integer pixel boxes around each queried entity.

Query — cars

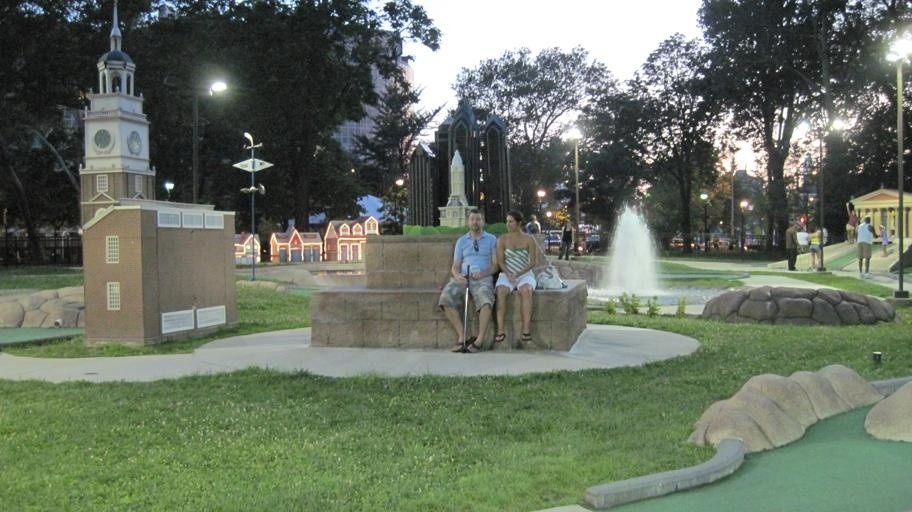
[543,231,601,253]
[672,238,778,252]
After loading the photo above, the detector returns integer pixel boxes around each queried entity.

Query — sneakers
[857,272,873,279]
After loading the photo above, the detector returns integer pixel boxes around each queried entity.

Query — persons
[845,202,859,245]
[877,224,888,247]
[854,216,879,279]
[784,224,801,271]
[526,214,541,235]
[493,209,538,344]
[437,209,499,354]
[808,226,821,268]
[557,220,574,261]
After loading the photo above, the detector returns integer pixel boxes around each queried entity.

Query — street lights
[537,189,546,230]
[795,119,843,274]
[164,67,236,206]
[559,126,583,256]
[885,38,911,298]
[700,193,749,257]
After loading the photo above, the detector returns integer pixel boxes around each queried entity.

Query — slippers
[522,332,532,341]
[495,333,506,342]
[451,336,483,353]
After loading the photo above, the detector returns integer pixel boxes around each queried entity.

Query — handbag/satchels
[537,266,562,289]
